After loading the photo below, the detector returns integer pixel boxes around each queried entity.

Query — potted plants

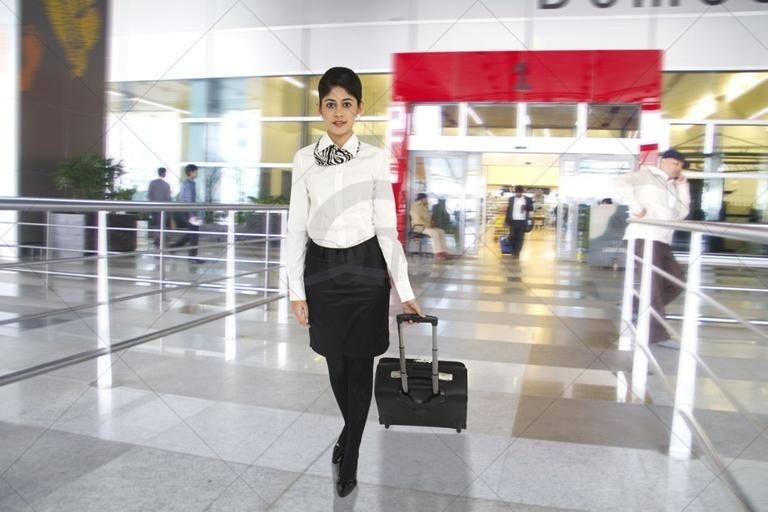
[43,152,136,258]
[230,186,292,243]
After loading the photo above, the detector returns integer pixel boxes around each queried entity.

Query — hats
[662,150,689,169]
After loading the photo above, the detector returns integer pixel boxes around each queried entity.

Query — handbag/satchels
[523,218,533,232]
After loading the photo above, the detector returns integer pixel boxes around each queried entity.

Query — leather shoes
[332,425,356,497]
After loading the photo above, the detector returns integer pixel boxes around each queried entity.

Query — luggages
[500,225,512,254]
[375,314,467,433]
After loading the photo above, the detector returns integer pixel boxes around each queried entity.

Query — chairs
[405,211,435,262]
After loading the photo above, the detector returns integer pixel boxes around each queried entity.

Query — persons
[409,192,450,262]
[505,186,533,258]
[285,66,426,500]
[601,198,616,205]
[432,193,460,250]
[168,165,205,265]
[691,210,711,253]
[614,150,693,350]
[146,168,172,250]
